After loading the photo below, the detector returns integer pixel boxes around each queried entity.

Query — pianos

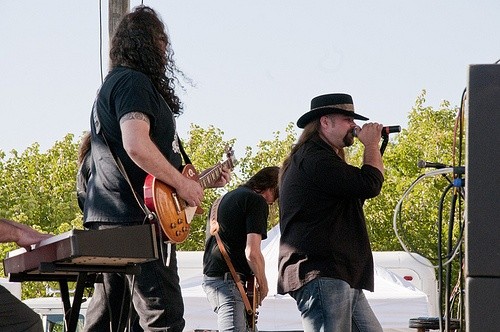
[2,223,159,332]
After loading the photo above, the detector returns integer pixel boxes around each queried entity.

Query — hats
[296,93,369,129]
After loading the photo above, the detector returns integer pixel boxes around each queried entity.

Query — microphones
[417,160,452,169]
[353,126,402,137]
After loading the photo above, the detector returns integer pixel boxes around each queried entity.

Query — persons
[0,219,55,332]
[277,93,383,332]
[82,3,232,332]
[77,132,92,212]
[203,166,280,332]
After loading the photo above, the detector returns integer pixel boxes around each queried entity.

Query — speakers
[465,64,500,332]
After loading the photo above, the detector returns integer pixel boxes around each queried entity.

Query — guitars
[246,275,261,332]
[144,146,238,244]
